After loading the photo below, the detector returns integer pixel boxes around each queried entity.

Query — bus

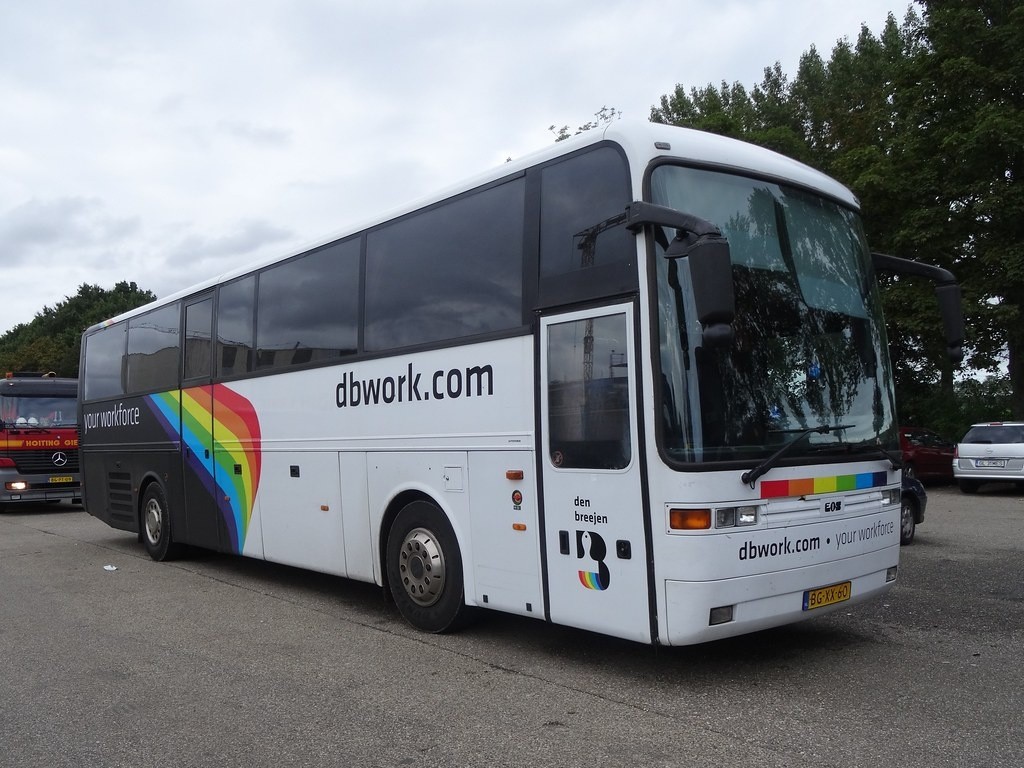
[76,119,967,650]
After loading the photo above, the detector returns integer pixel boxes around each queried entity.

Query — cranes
[572,212,627,381]
[124,323,247,345]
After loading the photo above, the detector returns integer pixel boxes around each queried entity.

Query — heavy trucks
[0,371,81,513]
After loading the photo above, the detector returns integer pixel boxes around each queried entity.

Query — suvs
[952,421,1024,492]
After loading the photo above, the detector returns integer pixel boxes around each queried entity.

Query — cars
[900,471,928,544]
[898,427,957,482]
